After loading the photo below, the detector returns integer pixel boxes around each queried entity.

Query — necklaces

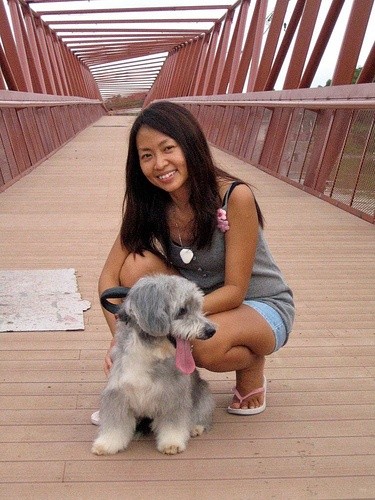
[171,202,199,265]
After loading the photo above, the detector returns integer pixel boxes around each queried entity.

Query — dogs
[90,273,214,455]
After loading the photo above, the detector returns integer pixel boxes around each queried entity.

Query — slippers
[222,357,270,417]
[91,410,101,427]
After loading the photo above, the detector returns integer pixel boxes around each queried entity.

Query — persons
[89,101,297,426]
